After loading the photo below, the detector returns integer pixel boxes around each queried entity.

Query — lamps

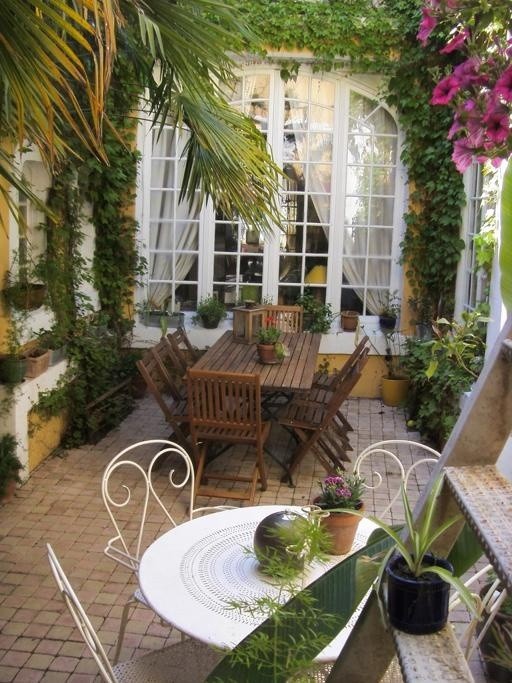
[304,263,327,302]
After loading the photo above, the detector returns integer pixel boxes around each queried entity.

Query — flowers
[314,465,371,515]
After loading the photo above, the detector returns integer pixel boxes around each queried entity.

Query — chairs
[346,437,444,529]
[41,537,230,683]
[98,437,242,669]
[308,557,509,683]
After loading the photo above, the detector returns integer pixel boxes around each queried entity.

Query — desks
[137,502,393,666]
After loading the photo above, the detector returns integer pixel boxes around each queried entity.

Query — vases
[311,495,365,557]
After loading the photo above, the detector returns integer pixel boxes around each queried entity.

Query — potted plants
[189,292,228,329]
[293,288,342,337]
[0,238,113,418]
[480,565,512,622]
[309,464,488,637]
[376,288,405,333]
[0,433,28,506]
[361,324,413,409]
